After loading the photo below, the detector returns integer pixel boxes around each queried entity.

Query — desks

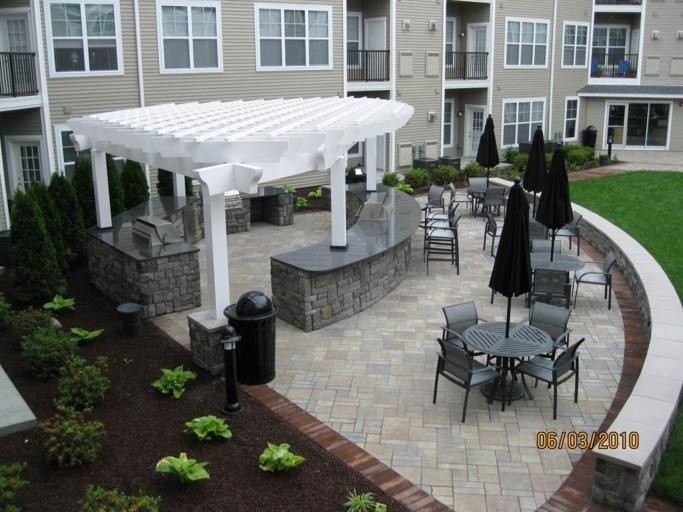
[464,322,554,411]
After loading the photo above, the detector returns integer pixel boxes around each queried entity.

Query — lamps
[70,51,79,65]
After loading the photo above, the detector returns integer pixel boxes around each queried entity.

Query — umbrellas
[476,114,499,190]
[523,124,548,218]
[534,145,574,263]
[487,177,532,341]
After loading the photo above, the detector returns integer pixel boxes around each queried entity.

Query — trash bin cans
[584,125,597,147]
[223,291,280,386]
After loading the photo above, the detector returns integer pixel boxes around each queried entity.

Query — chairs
[437,301,492,374]
[420,176,617,310]
[433,326,506,422]
[508,338,586,419]
[592,56,602,77]
[618,60,630,76]
[518,300,574,390]
